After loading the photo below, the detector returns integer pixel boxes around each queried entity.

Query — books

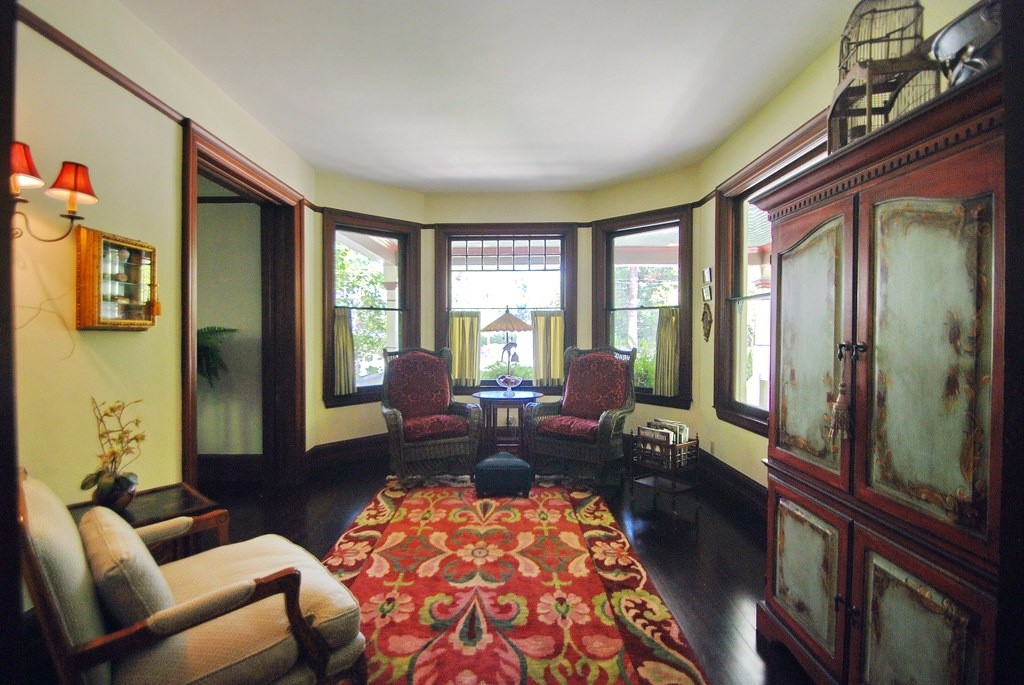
[646,418,689,466]
[637,427,673,467]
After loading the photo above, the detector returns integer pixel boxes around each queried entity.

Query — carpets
[322,472,711,685]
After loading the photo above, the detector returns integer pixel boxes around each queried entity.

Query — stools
[471,451,531,498]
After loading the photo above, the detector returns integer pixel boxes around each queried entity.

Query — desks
[474,391,544,456]
[64,478,231,565]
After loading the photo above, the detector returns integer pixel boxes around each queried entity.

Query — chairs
[378,345,483,474]
[17,467,369,685]
[526,345,637,485]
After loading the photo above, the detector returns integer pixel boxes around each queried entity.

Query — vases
[91,471,139,511]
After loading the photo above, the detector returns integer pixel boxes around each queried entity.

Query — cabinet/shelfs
[625,427,701,539]
[748,50,1024,684]
[73,224,157,330]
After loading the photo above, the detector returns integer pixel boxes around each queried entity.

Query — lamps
[10,141,98,243]
[479,304,536,397]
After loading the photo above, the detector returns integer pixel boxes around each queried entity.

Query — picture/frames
[702,267,711,284]
[702,286,712,301]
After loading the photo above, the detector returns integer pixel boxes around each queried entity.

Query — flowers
[80,396,150,491]
